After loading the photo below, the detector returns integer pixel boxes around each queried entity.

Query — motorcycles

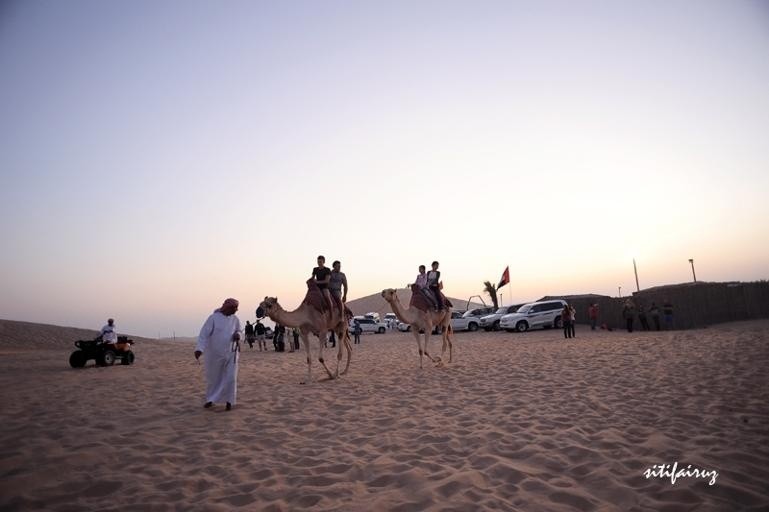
[70,336,135,367]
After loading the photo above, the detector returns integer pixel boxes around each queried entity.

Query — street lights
[689,259,698,285]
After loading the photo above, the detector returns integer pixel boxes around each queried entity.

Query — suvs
[348,300,577,335]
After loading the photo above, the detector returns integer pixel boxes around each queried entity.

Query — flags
[497,267,509,288]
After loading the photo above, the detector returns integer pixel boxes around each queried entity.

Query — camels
[256,277,355,385]
[382,284,452,370]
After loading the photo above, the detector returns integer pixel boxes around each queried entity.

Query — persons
[245,321,254,348]
[353,319,361,344]
[561,305,572,338]
[622,299,674,333]
[569,304,576,338]
[95,319,122,357]
[194,299,242,410]
[328,261,347,322]
[272,323,300,352]
[330,330,335,347]
[411,265,438,313]
[427,262,442,311]
[590,304,597,329]
[253,320,268,351]
[311,256,334,321]
